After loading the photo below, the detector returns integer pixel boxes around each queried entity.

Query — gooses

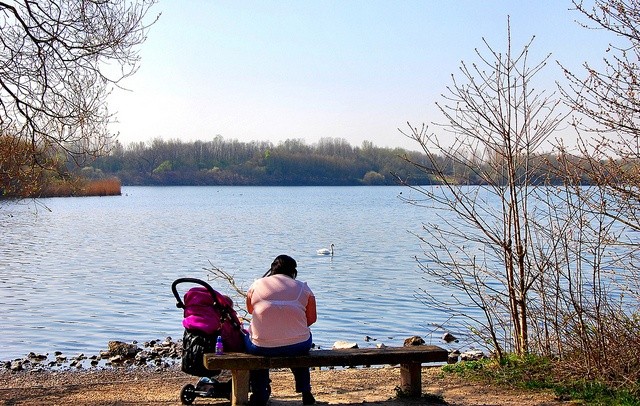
[315,244,335,254]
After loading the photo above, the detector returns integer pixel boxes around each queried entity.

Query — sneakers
[303,392,315,406]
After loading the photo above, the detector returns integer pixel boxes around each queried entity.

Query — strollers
[170,277,272,405]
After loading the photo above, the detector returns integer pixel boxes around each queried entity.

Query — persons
[246,253,317,406]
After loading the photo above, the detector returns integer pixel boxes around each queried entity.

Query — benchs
[203,343,448,404]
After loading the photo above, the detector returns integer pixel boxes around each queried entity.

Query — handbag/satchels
[182,309,226,377]
